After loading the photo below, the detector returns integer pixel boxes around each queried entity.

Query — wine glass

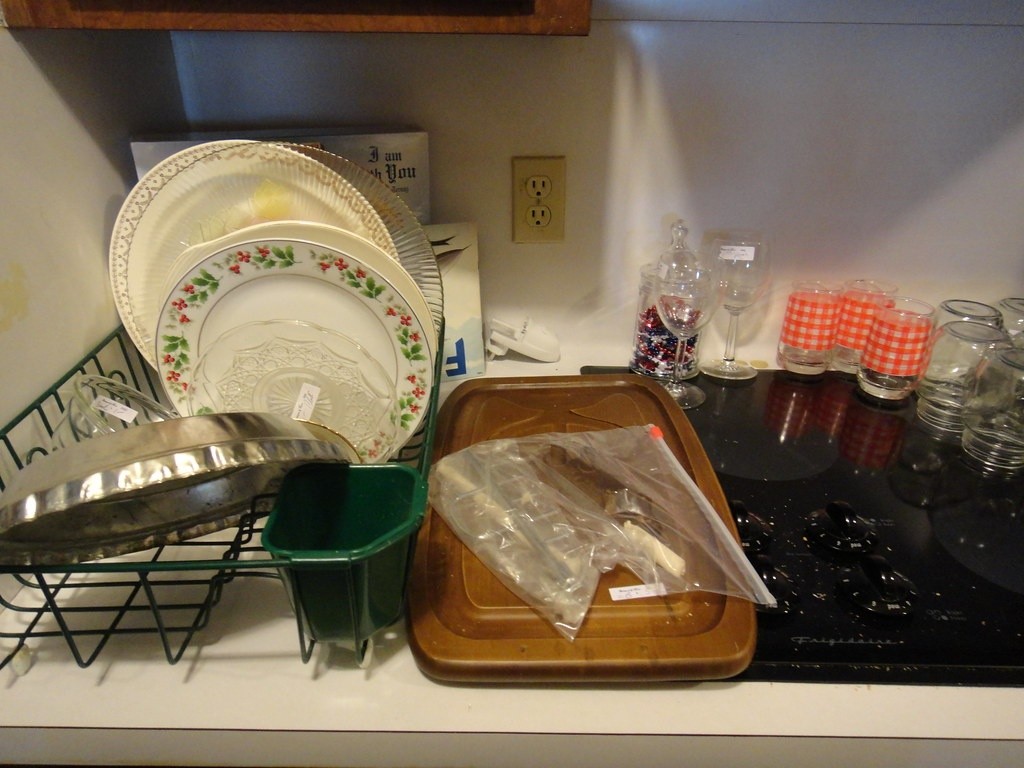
[657,262,724,411]
[699,238,772,381]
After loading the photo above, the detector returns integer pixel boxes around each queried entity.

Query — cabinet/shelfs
[0,310,446,670]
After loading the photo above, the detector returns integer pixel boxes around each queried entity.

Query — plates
[110,142,437,463]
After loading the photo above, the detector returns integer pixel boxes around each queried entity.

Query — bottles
[861,295,934,401]
[829,279,884,381]
[766,374,903,475]
[774,277,842,376]
[916,294,1024,470]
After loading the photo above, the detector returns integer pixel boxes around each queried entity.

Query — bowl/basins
[50,376,177,463]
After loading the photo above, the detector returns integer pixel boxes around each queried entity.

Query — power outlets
[512,155,567,245]
